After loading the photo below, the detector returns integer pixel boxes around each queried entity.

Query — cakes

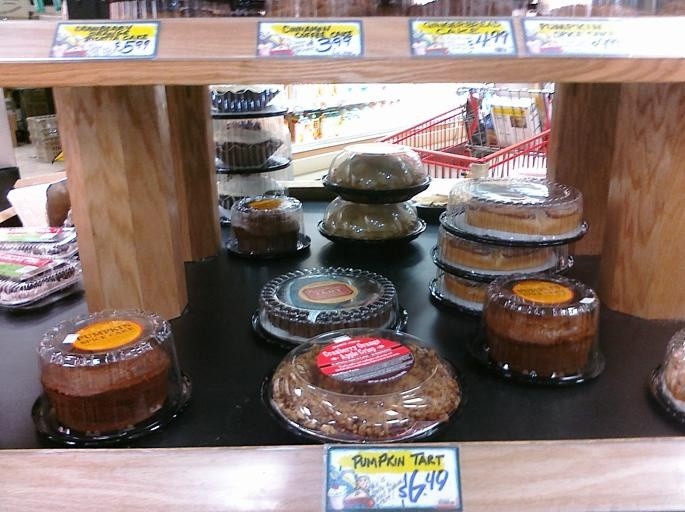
[482,284,590,374]
[469,194,581,235]
[333,152,425,189]
[444,273,488,303]
[234,210,299,252]
[326,203,419,240]
[440,234,550,271]
[659,342,685,408]
[215,122,283,166]
[41,345,171,434]
[218,172,283,219]
[267,277,391,339]
[274,339,461,436]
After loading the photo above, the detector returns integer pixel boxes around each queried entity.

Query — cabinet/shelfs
[210,107,292,226]
[0,0,685,512]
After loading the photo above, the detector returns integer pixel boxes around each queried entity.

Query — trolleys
[380,87,554,179]
